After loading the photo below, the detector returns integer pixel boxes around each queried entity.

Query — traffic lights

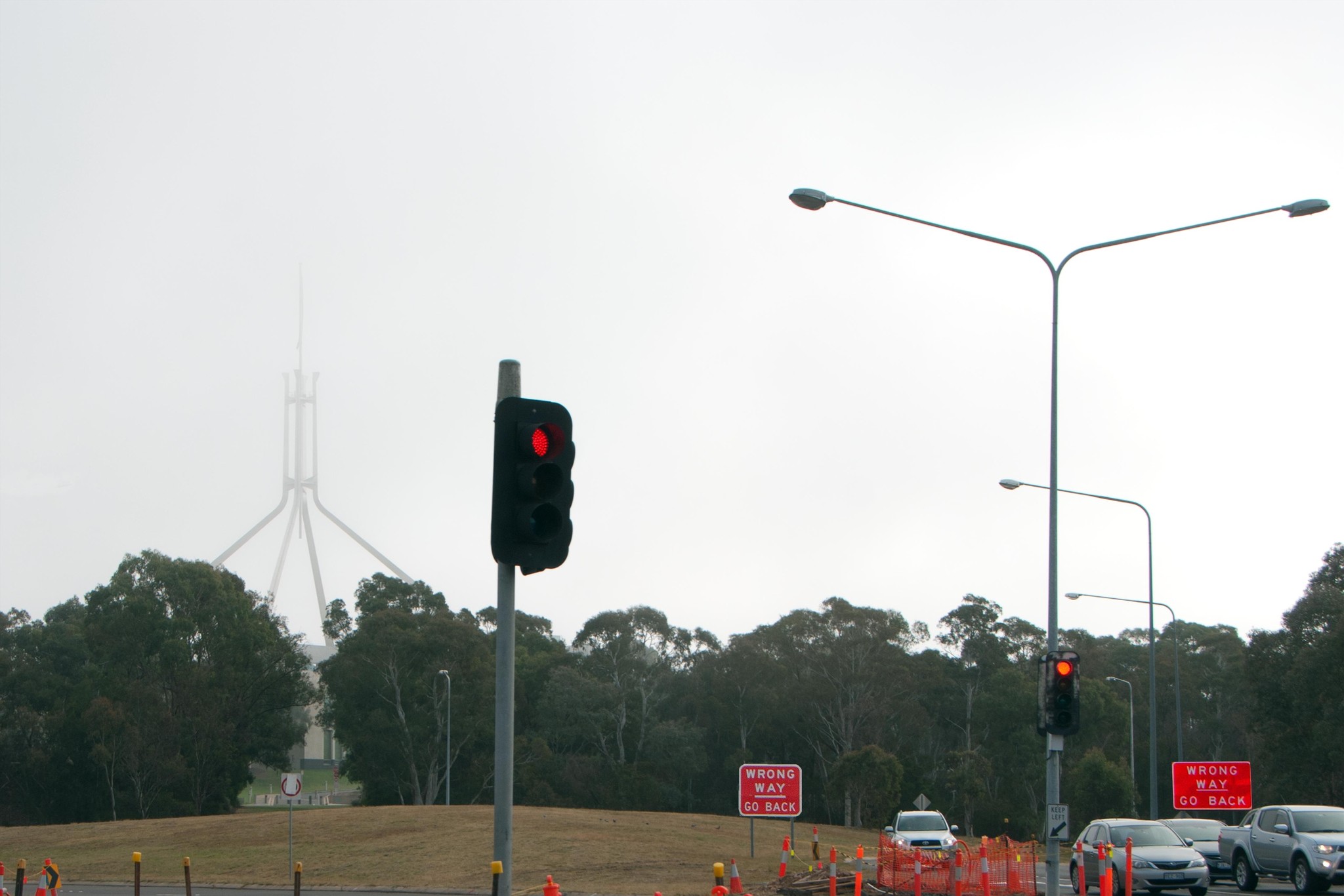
[491,394,573,573]
[1045,650,1080,734]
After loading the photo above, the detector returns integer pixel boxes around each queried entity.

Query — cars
[1068,818,1210,896]
[1155,819,1235,885]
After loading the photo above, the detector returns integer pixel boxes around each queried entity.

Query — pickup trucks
[1219,804,1344,894]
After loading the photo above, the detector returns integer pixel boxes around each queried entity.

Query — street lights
[1065,591,1188,820]
[788,186,1328,896]
[438,669,451,806]
[997,479,1158,819]
[1105,675,1136,811]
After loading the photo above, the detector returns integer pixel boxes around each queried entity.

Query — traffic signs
[739,762,803,817]
[1172,761,1253,810]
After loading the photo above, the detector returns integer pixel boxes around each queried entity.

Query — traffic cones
[725,859,747,896]
[35,869,46,896]
[46,859,57,896]
[0,861,4,896]
